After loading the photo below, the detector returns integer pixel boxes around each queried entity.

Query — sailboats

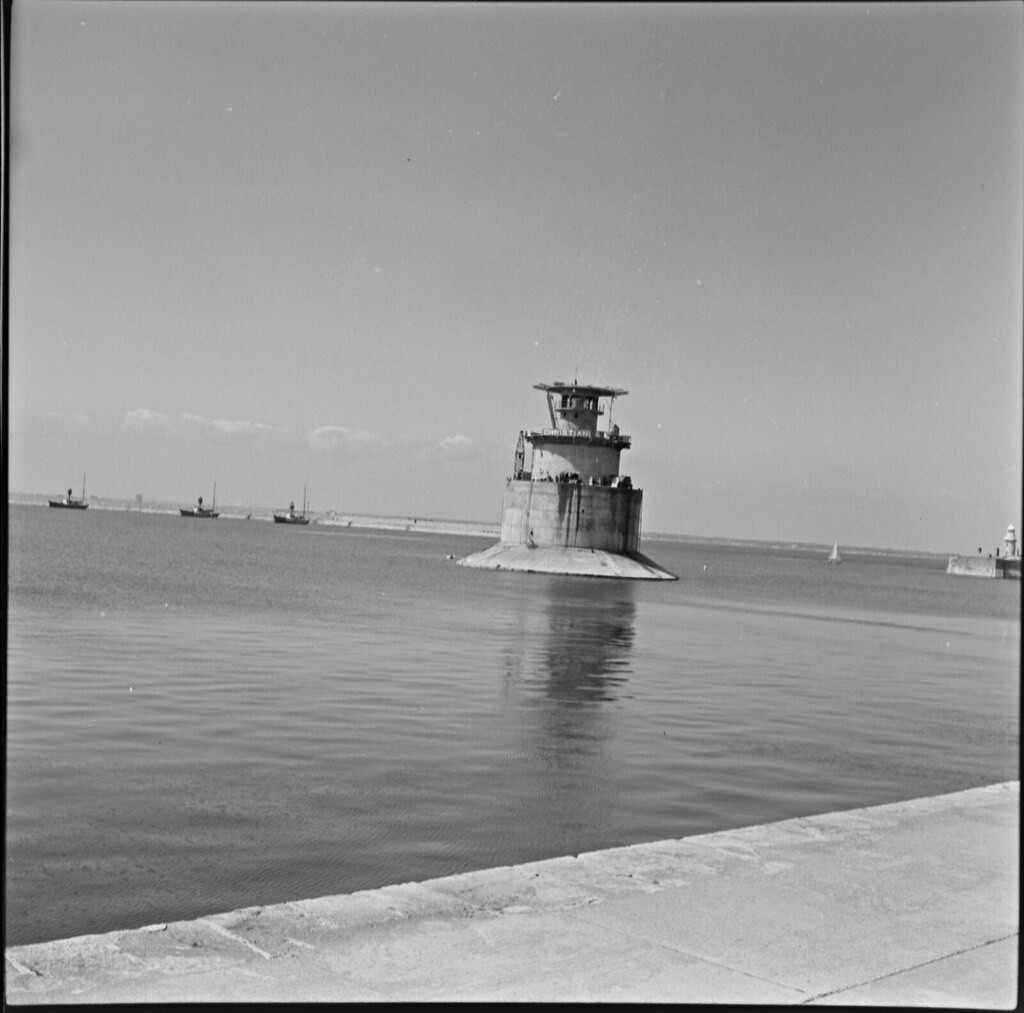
[828,540,842,565]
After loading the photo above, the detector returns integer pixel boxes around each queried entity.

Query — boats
[180,481,219,518]
[274,485,310,526]
[48,474,89,510]
